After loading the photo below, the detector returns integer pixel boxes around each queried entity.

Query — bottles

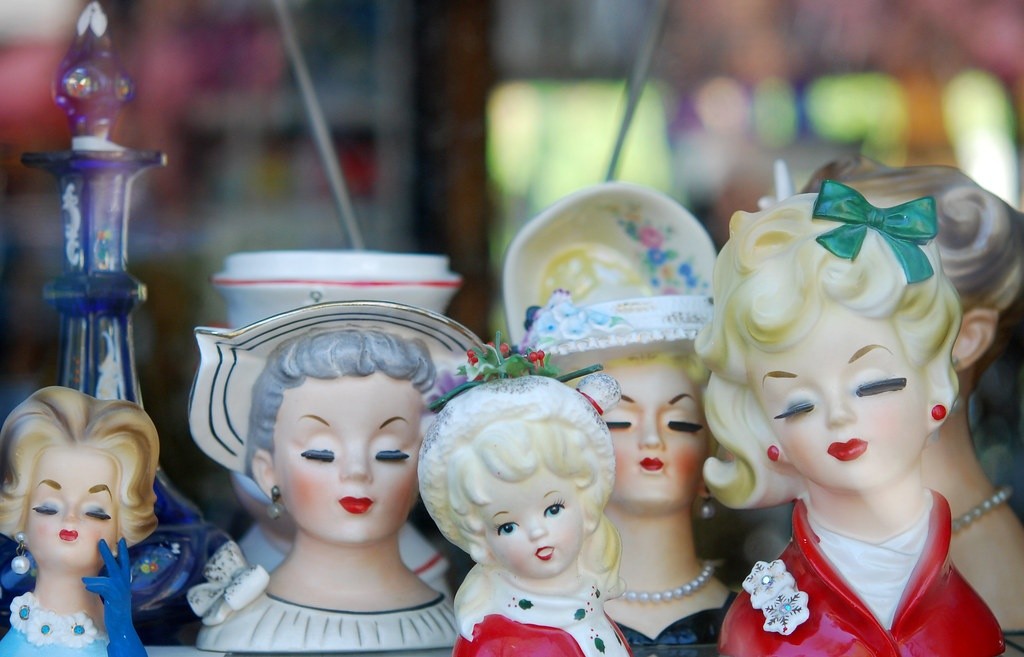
[14,0,242,623]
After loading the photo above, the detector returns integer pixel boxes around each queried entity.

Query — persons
[0,386,164,657]
[180,296,489,648]
[679,184,1012,657]
[408,333,632,655]
[510,288,751,650]
[799,152,1023,636]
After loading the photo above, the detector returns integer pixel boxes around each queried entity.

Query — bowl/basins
[501,181,720,351]
